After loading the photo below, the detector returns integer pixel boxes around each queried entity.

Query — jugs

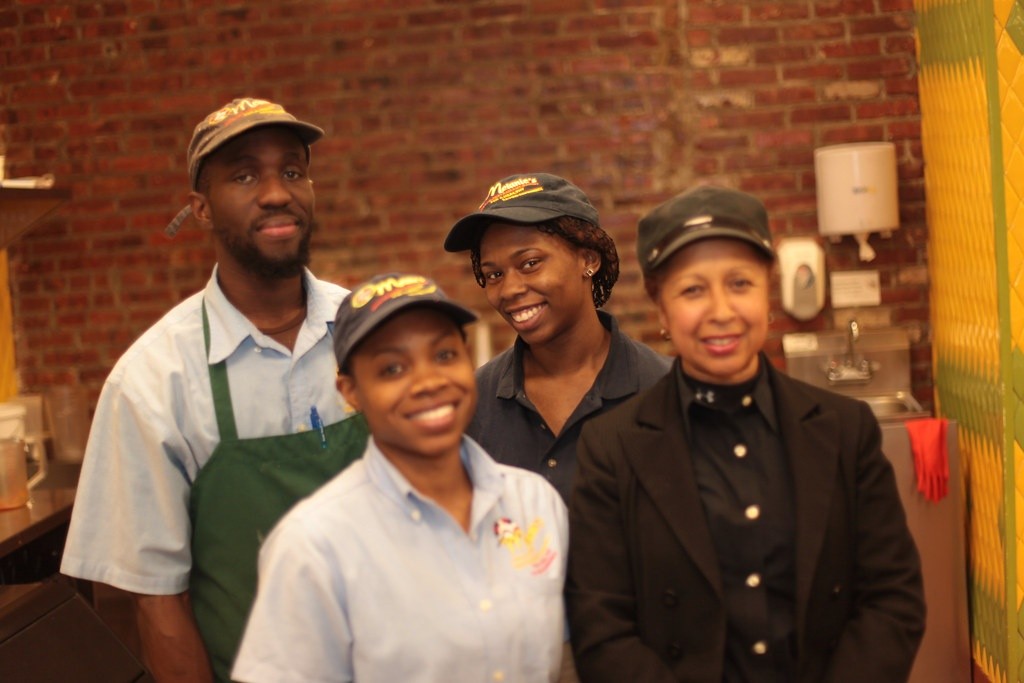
[0,395,43,460]
[0,419,47,510]
[46,384,92,463]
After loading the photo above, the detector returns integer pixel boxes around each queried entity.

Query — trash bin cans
[0,573,159,683]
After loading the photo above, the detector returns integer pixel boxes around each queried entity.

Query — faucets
[845,318,860,369]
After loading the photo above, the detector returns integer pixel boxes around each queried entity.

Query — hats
[332,274,478,374]
[186,97,326,190]
[444,172,601,253]
[635,183,777,279]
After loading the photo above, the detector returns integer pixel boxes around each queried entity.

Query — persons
[230,273,586,682]
[59,96,372,683]
[569,185,929,683]
[443,172,672,485]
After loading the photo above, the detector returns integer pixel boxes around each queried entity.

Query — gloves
[904,416,950,504]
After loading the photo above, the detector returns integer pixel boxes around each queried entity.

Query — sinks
[854,391,925,417]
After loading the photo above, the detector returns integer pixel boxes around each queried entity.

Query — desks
[0,453,145,683]
